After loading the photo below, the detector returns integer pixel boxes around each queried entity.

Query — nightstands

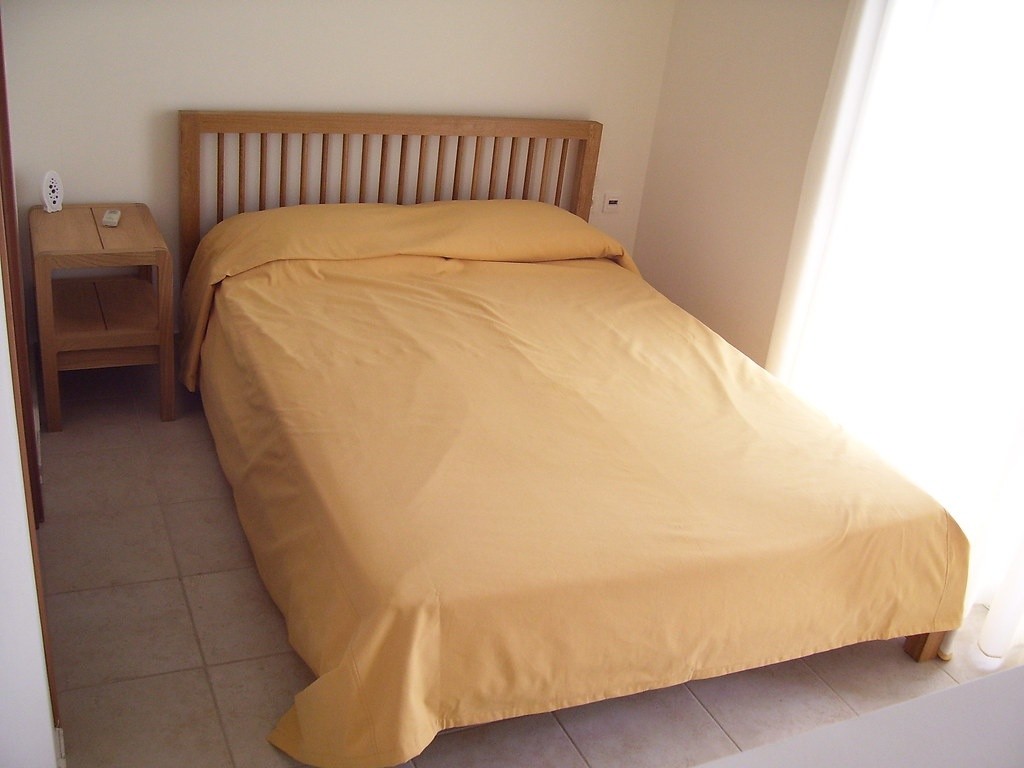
[29,203,177,428]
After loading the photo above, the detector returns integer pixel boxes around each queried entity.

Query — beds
[174,107,973,768]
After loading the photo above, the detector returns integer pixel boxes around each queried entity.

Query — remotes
[102,209,121,228]
